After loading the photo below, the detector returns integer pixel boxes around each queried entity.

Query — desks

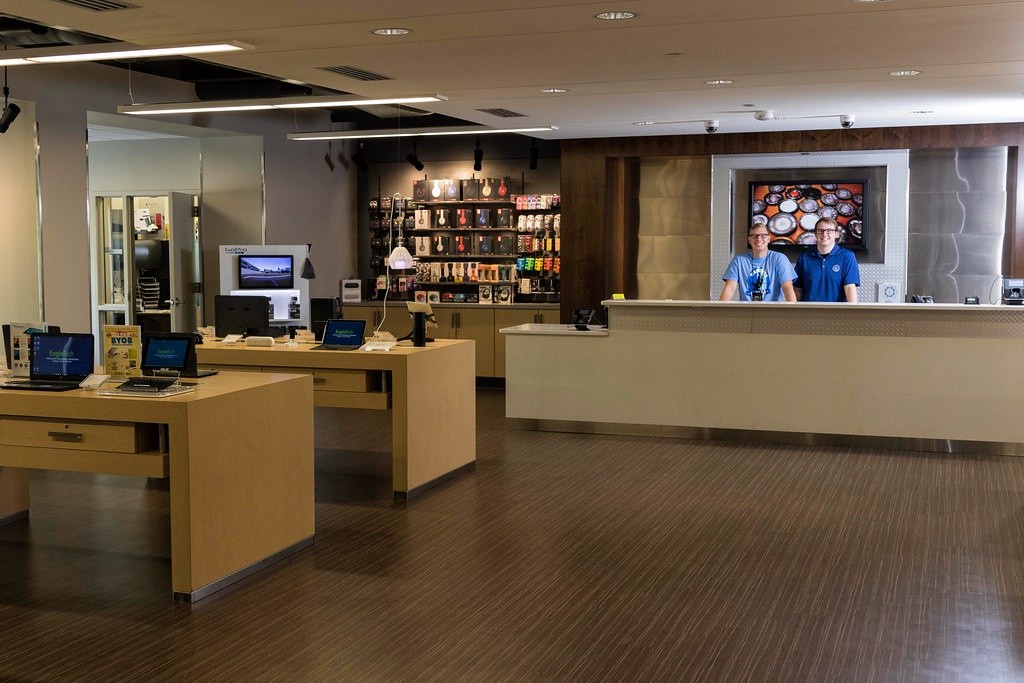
[195,336,476,505]
[0,371,315,604]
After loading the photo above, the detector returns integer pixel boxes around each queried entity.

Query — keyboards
[115,377,179,390]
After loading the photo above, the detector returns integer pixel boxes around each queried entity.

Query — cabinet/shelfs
[340,172,560,377]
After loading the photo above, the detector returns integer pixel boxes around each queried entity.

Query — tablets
[140,336,193,371]
[406,301,440,328]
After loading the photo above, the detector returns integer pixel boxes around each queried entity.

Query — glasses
[748,233,769,238]
[816,229,837,234]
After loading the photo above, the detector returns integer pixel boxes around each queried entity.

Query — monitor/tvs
[238,254,294,289]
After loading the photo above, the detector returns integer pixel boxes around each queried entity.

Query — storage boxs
[412,176,515,306]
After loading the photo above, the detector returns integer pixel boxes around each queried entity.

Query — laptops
[310,319,367,351]
[0,332,95,392]
[142,332,219,379]
[214,294,285,338]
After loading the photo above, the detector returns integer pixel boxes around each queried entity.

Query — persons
[793,217,860,302]
[719,223,798,303]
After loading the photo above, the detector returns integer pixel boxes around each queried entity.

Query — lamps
[404,139,427,171]
[472,133,487,172]
[351,140,370,172]
[528,136,540,170]
[358,192,414,350]
[116,62,449,115]
[0,40,265,66]
[0,40,22,134]
[286,109,560,141]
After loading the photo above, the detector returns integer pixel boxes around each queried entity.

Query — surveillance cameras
[706,119,719,134]
[840,115,855,128]
